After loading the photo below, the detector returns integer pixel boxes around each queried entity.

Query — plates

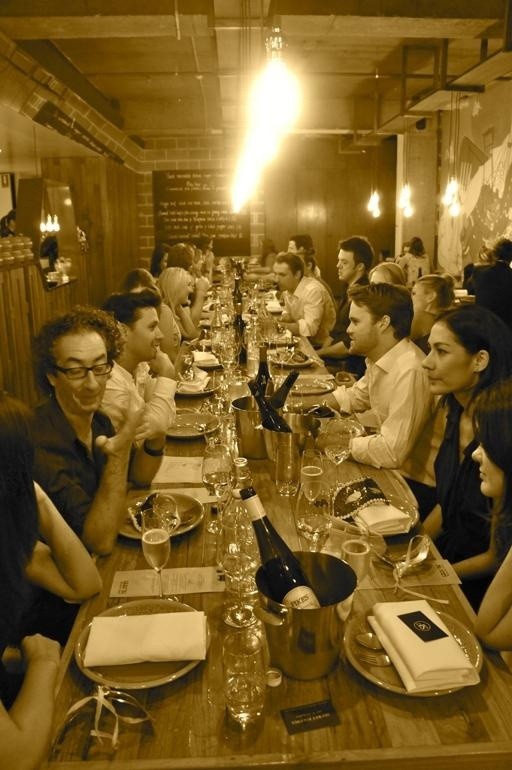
[118,489,207,541]
[267,348,319,369]
[314,418,367,444]
[331,484,420,536]
[74,600,212,691]
[280,374,337,396]
[166,408,223,438]
[343,602,485,698]
[174,371,224,394]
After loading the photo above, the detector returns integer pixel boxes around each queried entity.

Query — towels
[330,481,410,539]
[178,367,212,392]
[368,597,481,696]
[218,315,230,326]
[203,301,212,313]
[266,301,283,313]
[82,608,210,670]
[199,319,212,327]
[192,351,220,367]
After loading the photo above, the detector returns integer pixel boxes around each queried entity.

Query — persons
[315,236,376,383]
[246,238,277,275]
[201,237,215,273]
[23,307,168,555]
[289,282,449,521]
[420,305,512,612]
[188,248,204,279]
[166,243,210,328]
[258,250,336,367]
[0,208,19,240]
[298,254,338,311]
[411,273,455,356]
[399,237,430,288]
[157,267,198,367]
[1,389,105,770]
[288,234,321,279]
[99,288,178,449]
[119,268,181,377]
[466,238,512,311]
[368,261,407,287]
[463,244,494,288]
[151,243,171,282]
[451,381,512,676]
[395,242,412,265]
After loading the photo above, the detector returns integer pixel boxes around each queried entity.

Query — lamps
[368,138,382,217]
[232,0,299,213]
[442,93,462,219]
[40,182,60,233]
[400,121,415,219]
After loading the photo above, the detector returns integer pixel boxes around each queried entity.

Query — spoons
[166,420,208,433]
[355,633,388,651]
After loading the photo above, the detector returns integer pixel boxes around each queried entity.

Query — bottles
[235,263,243,279]
[256,346,271,395]
[240,487,323,608]
[233,302,246,362]
[233,278,243,302]
[247,381,293,433]
[270,371,299,408]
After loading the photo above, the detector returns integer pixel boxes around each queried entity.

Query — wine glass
[322,416,353,491]
[140,507,178,600]
[301,449,325,518]
[201,443,238,534]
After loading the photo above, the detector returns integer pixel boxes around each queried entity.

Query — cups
[274,446,303,500]
[294,481,334,554]
[246,345,260,384]
[340,538,373,586]
[221,633,270,728]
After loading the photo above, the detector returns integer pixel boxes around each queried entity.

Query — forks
[359,653,391,667]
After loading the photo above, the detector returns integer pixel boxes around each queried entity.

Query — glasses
[50,362,117,381]
